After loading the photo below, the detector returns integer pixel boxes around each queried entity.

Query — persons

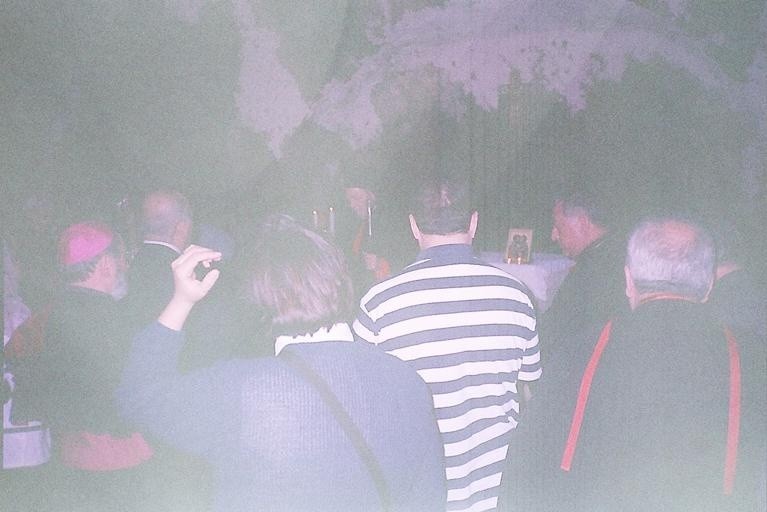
[354,179,543,512]
[706,214,765,338]
[120,177,201,359]
[338,187,403,284]
[117,214,446,511]
[504,192,630,388]
[509,235,528,260]
[2,222,159,511]
[496,213,766,512]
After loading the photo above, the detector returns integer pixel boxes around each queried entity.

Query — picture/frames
[506,228,532,264]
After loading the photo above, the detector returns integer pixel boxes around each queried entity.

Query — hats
[56,222,113,262]
